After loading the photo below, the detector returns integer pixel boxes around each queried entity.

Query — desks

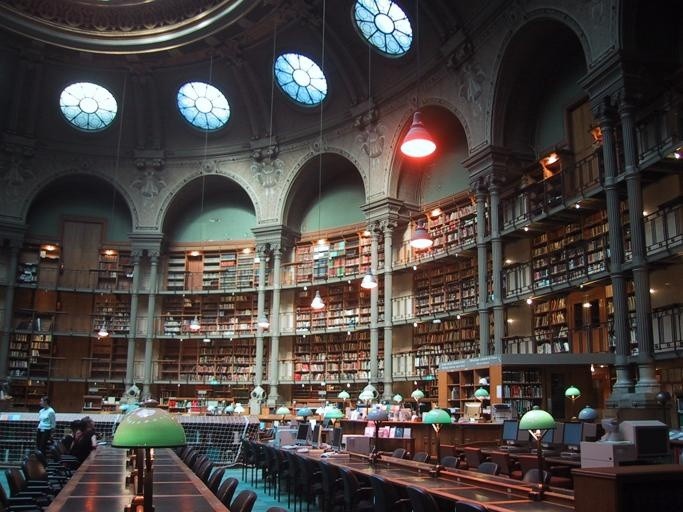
[570,463,683,512]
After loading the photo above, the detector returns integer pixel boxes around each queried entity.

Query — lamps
[400,0,437,160]
[408,159,434,250]
[190,50,213,330]
[98,70,128,338]
[578,405,597,425]
[655,390,672,425]
[257,0,281,328]
[310,1,326,310]
[361,44,378,289]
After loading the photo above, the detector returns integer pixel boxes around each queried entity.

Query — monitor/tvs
[502,420,519,441]
[540,429,553,444]
[296,422,343,452]
[563,422,584,451]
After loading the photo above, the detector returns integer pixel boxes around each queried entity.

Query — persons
[214,400,451,422]
[37,396,55,452]
[70,416,102,466]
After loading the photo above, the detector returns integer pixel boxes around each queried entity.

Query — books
[502,370,542,384]
[11,264,51,376]
[93,255,130,331]
[163,252,269,383]
[413,204,508,383]
[295,230,383,383]
[530,206,641,356]
